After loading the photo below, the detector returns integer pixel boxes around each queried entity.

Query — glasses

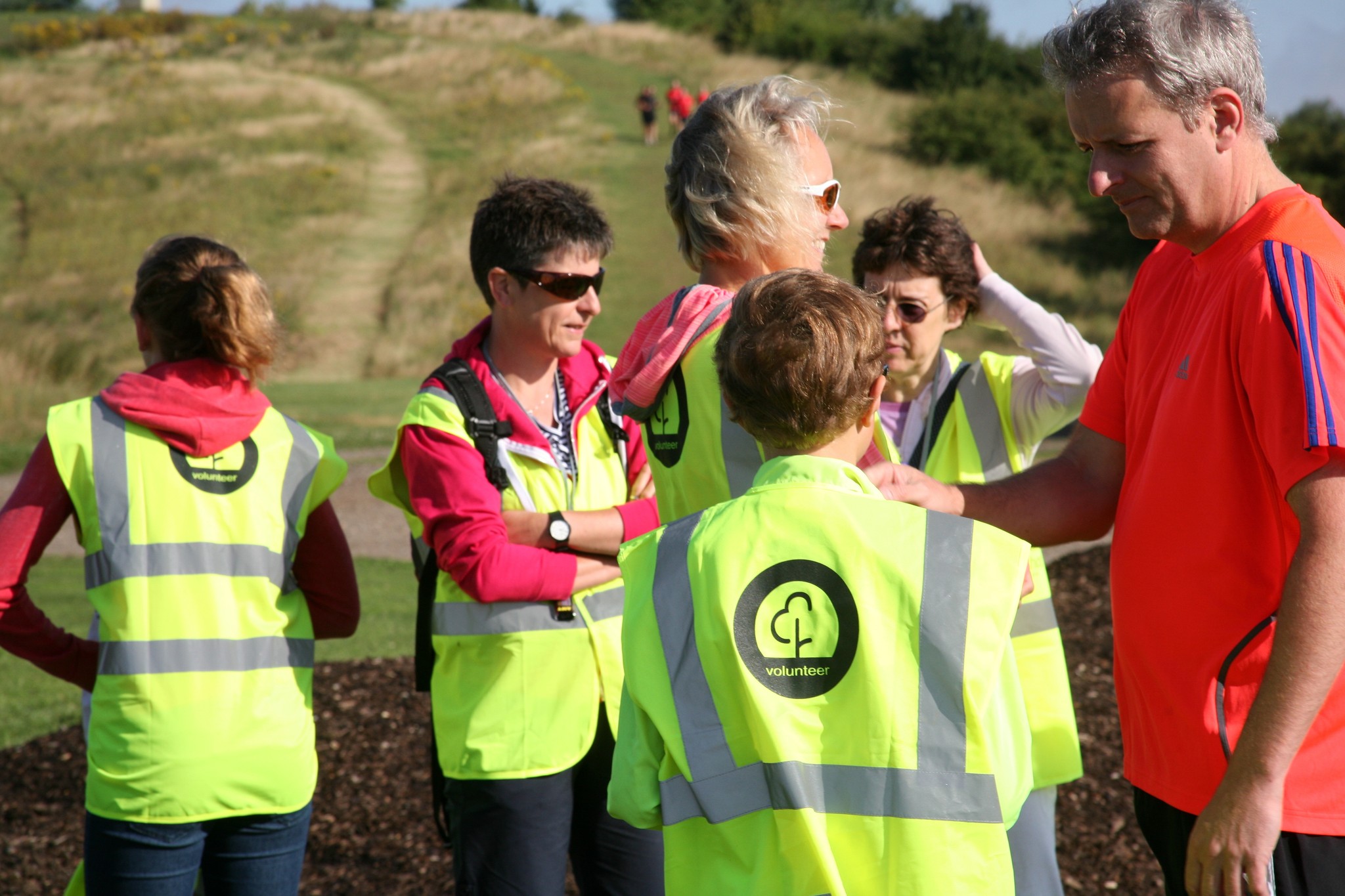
[505,263,604,300]
[781,180,841,214]
[870,291,960,323]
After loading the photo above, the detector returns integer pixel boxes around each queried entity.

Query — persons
[638,80,710,146]
[366,176,660,894]
[869,3,1345,895]
[852,192,1106,483]
[608,269,1086,894]
[0,238,362,895]
[607,75,901,526]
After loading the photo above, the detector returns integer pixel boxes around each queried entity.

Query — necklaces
[526,383,555,414]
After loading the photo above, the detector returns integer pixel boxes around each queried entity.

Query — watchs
[549,511,570,551]
